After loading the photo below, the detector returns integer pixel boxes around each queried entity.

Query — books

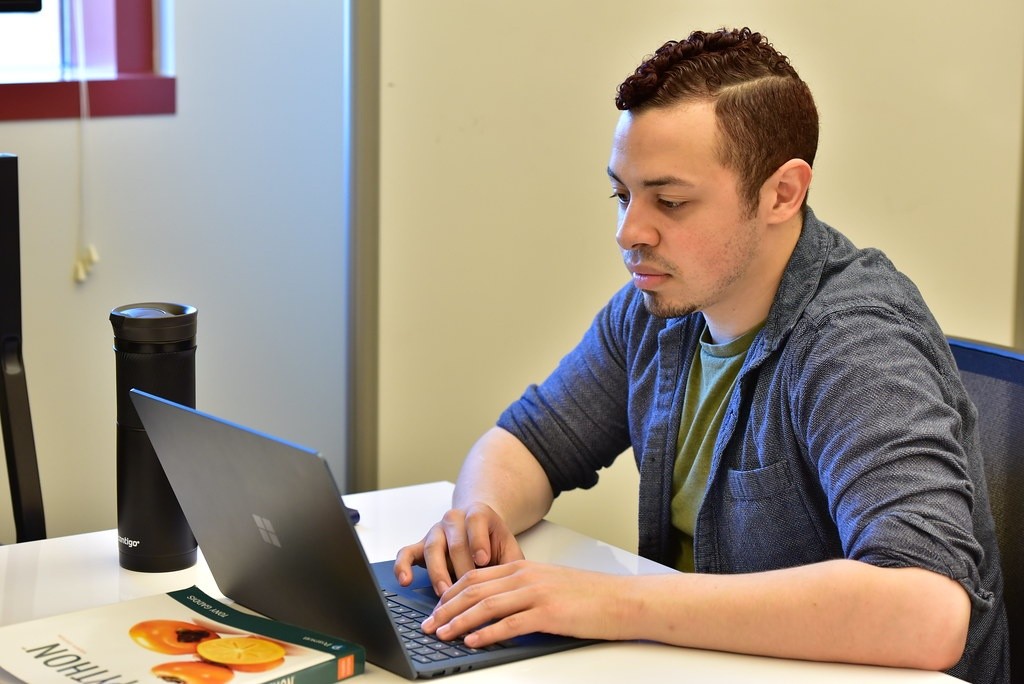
[0,585,365,684]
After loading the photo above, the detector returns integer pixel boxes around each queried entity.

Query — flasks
[111,302,199,572]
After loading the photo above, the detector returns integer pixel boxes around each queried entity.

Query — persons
[394,27,1012,684]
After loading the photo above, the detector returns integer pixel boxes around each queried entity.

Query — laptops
[129,387,605,681]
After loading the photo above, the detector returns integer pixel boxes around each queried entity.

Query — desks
[0,478,971,684]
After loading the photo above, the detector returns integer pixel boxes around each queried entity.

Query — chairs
[947,335,1024,684]
